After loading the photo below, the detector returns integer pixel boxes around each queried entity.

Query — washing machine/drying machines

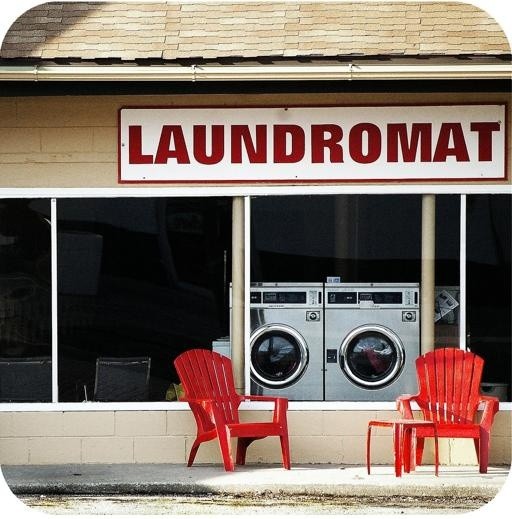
[324,281,421,402]
[229,281,324,401]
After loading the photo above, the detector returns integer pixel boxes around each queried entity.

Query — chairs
[173,348,292,472]
[395,346,499,473]
[0,353,152,403]
